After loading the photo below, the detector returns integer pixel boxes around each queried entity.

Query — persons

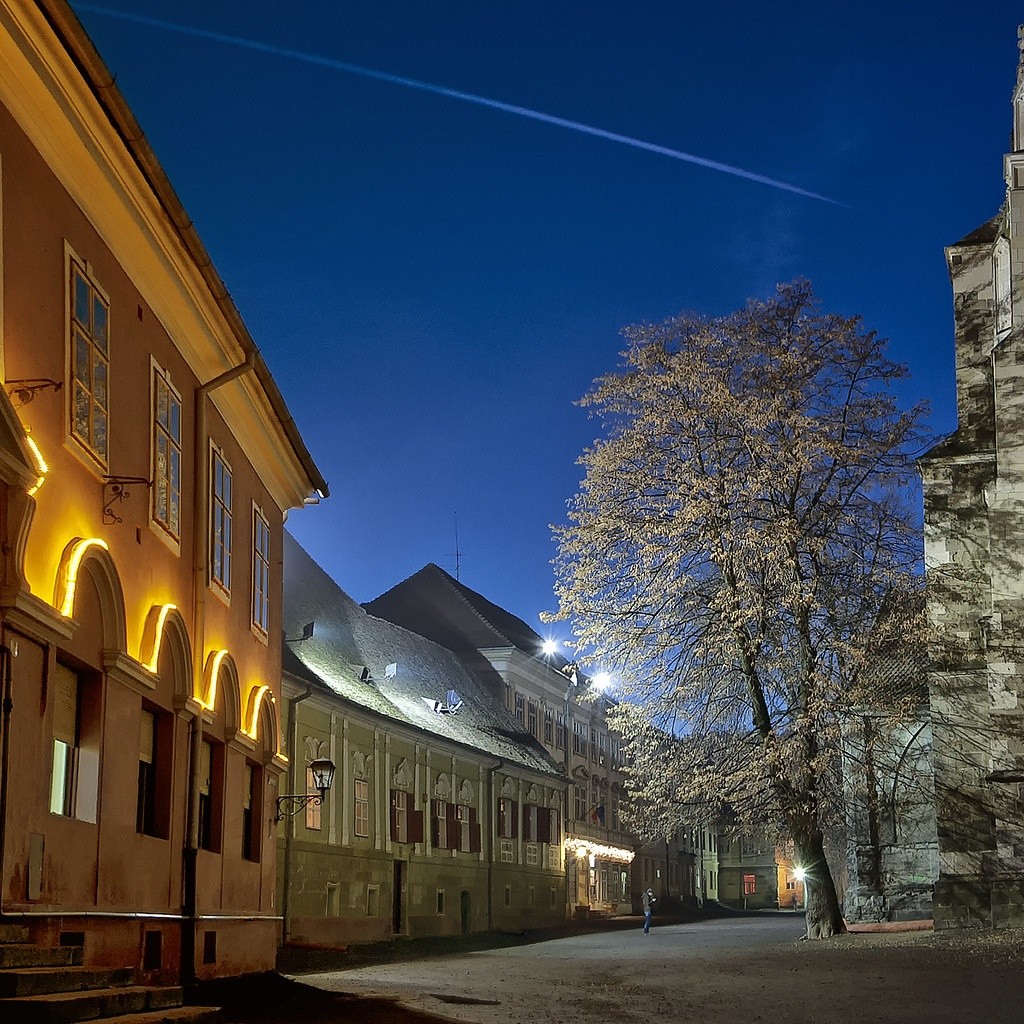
[641,886,657,936]
[792,892,798,911]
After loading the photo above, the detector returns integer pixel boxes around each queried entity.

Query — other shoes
[644,933,650,935]
